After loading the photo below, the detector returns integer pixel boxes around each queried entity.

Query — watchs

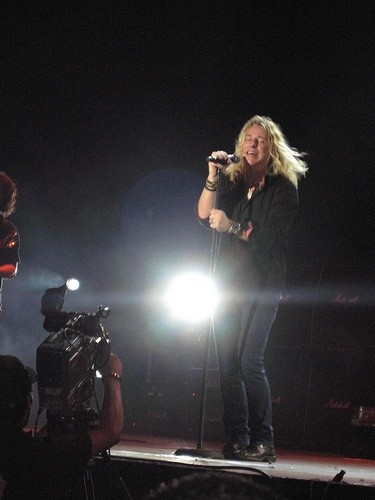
[228,218,241,237]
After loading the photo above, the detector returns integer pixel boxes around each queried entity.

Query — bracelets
[103,370,121,382]
[204,179,220,191]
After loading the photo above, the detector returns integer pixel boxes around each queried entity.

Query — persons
[196,113,310,463]
[0,172,123,500]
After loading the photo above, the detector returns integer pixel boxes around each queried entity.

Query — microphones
[207,154,240,163]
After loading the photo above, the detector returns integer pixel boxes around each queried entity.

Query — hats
[0,356,37,396]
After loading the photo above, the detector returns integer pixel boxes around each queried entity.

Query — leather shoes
[221,445,248,457]
[233,443,277,462]
